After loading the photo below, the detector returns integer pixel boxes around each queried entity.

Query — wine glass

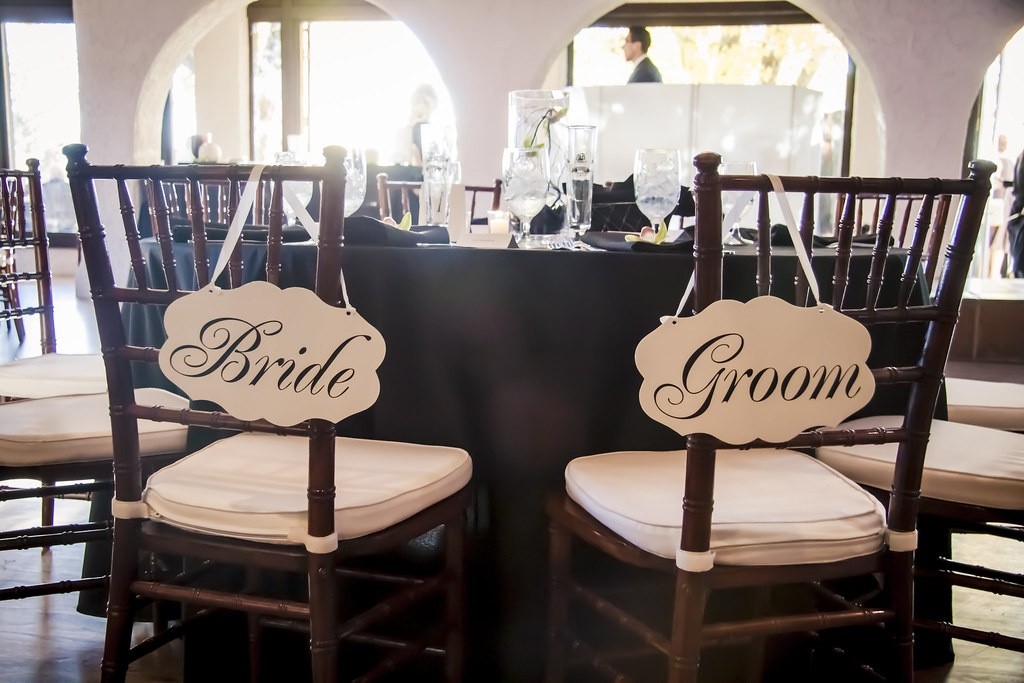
[272,151,313,228]
[718,161,759,246]
[633,148,681,235]
[501,147,551,249]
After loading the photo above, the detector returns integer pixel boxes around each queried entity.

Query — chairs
[541,152,1024,683]
[0,146,506,682]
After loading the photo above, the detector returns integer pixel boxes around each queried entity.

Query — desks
[137,165,422,236]
[78,232,950,683]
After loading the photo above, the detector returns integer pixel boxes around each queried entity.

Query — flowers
[625,222,668,245]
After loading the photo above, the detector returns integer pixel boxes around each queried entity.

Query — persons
[622,24,663,85]
[408,92,433,162]
[984,135,1024,279]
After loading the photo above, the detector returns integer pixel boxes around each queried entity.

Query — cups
[487,210,510,234]
[565,124,597,230]
[420,123,458,227]
[317,141,367,218]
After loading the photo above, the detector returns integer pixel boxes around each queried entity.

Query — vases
[508,88,578,249]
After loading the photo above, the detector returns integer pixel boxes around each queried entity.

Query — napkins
[168,217,312,243]
[733,224,793,246]
[853,233,894,247]
[574,229,696,252]
[343,215,452,246]
[566,176,694,228]
[531,204,566,235]
[814,233,837,248]
